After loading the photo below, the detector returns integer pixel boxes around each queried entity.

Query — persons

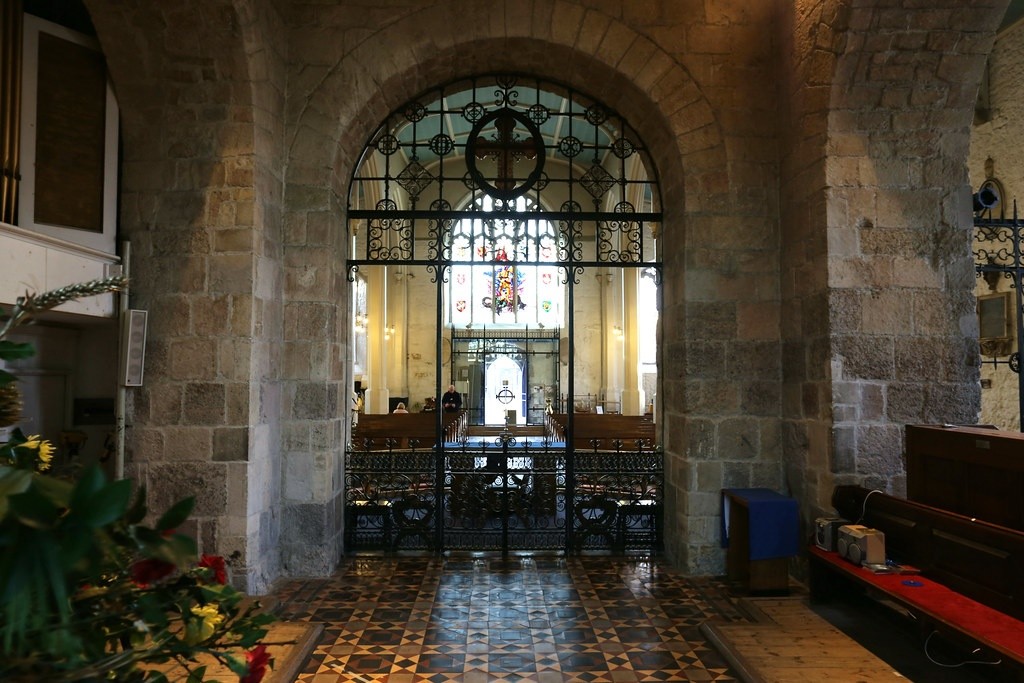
[393,402,408,413]
[442,385,462,412]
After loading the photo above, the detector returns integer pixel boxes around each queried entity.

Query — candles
[361,375,368,387]
[505,408,508,416]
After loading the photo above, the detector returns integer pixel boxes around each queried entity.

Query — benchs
[545,412,656,450]
[805,484,1024,683]
[354,410,469,449]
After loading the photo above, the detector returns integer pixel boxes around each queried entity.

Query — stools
[345,500,393,548]
[615,499,658,548]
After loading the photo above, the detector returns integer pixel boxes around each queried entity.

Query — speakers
[815,517,852,552]
[838,526,886,566]
[120,309,148,386]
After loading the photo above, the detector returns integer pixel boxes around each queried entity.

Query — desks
[720,486,800,597]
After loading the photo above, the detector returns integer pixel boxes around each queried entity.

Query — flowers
[0,273,286,683]
